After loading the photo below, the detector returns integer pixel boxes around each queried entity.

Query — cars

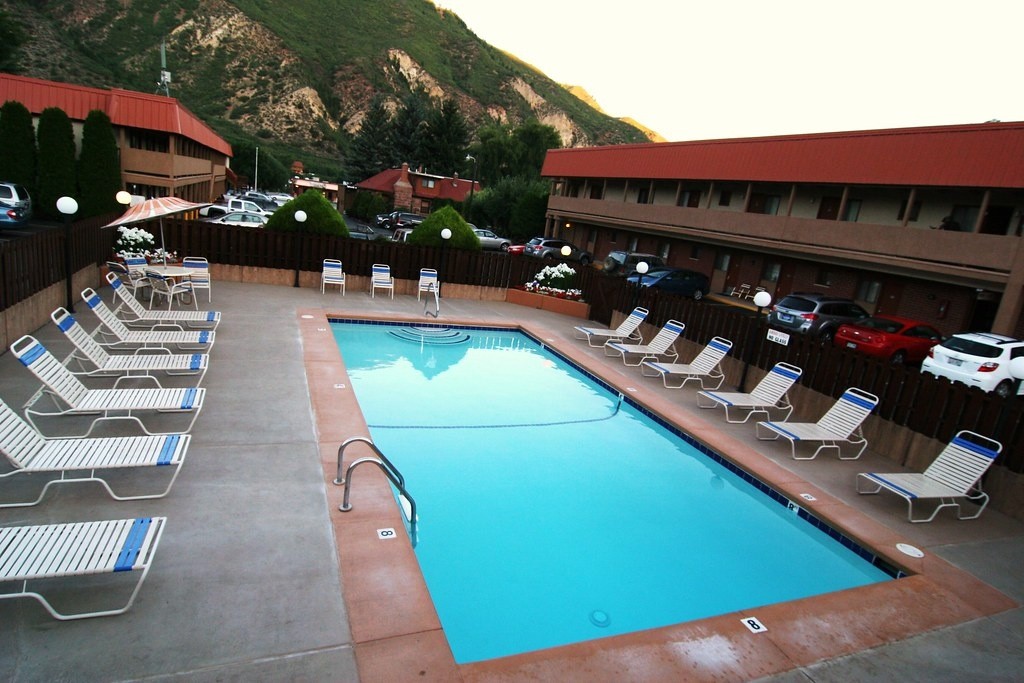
[222,190,283,212]
[467,223,478,230]
[767,291,872,350]
[198,212,269,228]
[268,194,295,202]
[0,201,26,240]
[472,230,513,252]
[836,315,950,371]
[376,211,427,242]
[349,224,380,241]
[920,332,1024,401]
[626,266,711,301]
[507,245,526,256]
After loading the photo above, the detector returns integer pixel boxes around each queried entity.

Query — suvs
[601,249,667,279]
[0,181,32,214]
[524,237,594,268]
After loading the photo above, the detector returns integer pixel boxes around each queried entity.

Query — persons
[220,194,224,199]
[101,197,213,270]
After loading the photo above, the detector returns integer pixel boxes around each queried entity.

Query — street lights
[465,154,477,222]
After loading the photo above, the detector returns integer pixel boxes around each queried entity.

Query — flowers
[523,262,587,303]
[112,225,178,264]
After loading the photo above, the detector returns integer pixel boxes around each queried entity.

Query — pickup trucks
[199,199,274,217]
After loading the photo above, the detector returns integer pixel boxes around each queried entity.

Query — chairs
[51,306,209,390]
[10,334,207,439]
[641,337,733,391]
[104,257,222,331]
[0,396,191,508]
[79,287,216,354]
[696,362,803,422]
[731,282,751,299]
[604,319,685,366]
[855,428,1002,523]
[745,285,766,301]
[574,307,649,348]
[369,264,394,302]
[756,387,879,460]
[416,267,440,303]
[320,259,345,296]
[0,516,167,620]
